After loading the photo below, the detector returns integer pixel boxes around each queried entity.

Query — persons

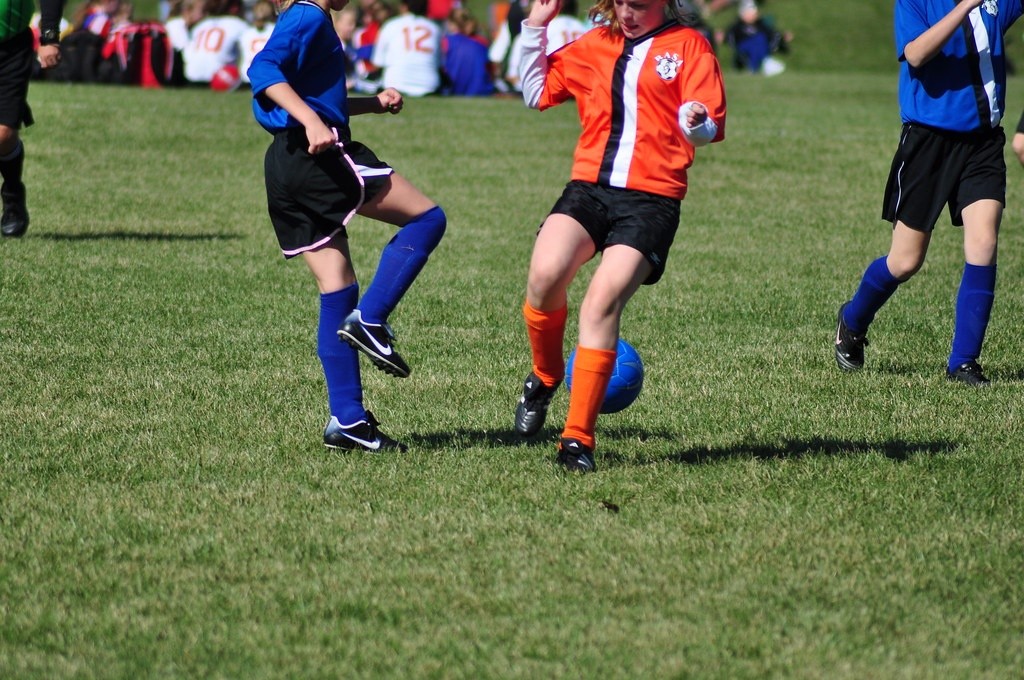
[246,0,461,451]
[515,1,726,473]
[30,0,794,99]
[834,0,1024,385]
[0,0,67,240]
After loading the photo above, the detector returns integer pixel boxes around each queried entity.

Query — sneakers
[515,373,563,436]
[836,301,869,373]
[557,438,597,474]
[946,360,989,385]
[324,410,408,455]
[337,308,410,378]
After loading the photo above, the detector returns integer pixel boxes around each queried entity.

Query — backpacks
[116,24,173,86]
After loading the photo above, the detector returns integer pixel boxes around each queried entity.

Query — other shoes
[0,183,29,238]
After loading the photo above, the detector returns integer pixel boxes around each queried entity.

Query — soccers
[565,339,644,414]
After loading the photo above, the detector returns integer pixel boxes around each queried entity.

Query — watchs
[45,29,57,40]
